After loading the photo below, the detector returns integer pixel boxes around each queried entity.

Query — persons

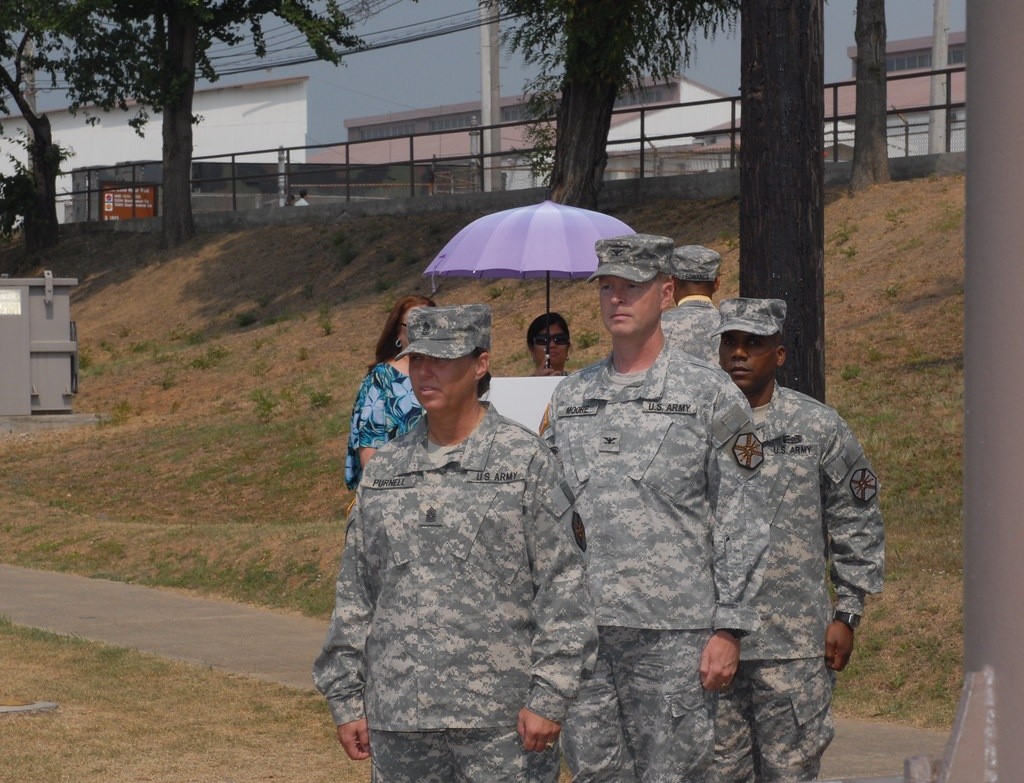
[539,233,771,783]
[710,298,885,783]
[344,245,721,492]
[284,189,309,206]
[312,303,599,783]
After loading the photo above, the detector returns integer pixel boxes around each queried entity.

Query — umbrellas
[422,199,637,369]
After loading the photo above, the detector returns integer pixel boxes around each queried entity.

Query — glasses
[532,333,569,346]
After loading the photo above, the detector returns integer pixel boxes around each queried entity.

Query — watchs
[721,628,748,640]
[835,611,860,629]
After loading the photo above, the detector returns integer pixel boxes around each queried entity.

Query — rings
[545,741,555,750]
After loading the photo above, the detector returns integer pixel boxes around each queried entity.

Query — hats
[671,245,720,281]
[711,298,787,340]
[586,233,674,283]
[395,304,493,359]
